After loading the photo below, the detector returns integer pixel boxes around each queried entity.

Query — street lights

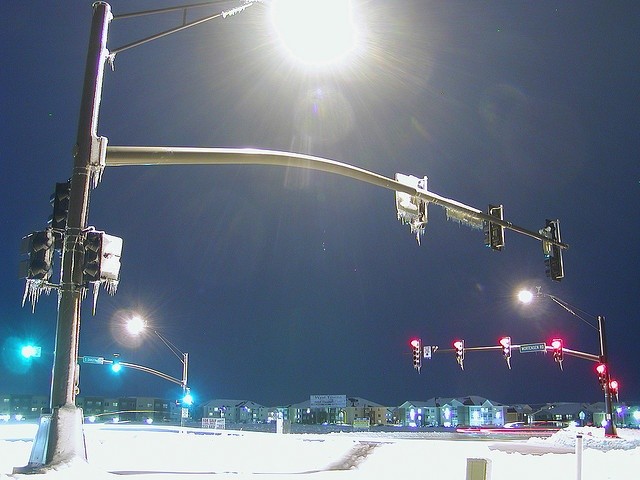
[127,318,188,427]
[517,286,621,439]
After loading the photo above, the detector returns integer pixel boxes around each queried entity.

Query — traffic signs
[82,356,103,365]
[519,343,547,354]
[27,0,358,466]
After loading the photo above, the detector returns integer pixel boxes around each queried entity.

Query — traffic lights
[551,339,565,362]
[499,336,512,358]
[539,219,565,282]
[409,339,423,369]
[112,354,120,372]
[596,365,607,391]
[23,229,55,290]
[84,229,124,286]
[609,380,619,400]
[484,205,506,253]
[45,183,76,250]
[452,339,464,362]
